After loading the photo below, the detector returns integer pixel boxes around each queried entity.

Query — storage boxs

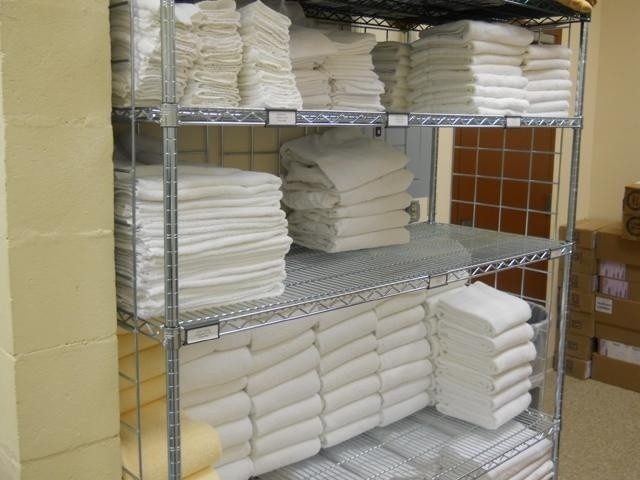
[550,181,640,394]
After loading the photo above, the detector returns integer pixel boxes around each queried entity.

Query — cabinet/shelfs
[111,0,592,480]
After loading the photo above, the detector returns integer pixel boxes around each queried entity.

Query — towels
[120,243,554,478]
[280,127,412,254]
[109,0,572,118]
[114,158,292,319]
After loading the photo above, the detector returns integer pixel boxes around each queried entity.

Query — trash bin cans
[528,301,551,410]
